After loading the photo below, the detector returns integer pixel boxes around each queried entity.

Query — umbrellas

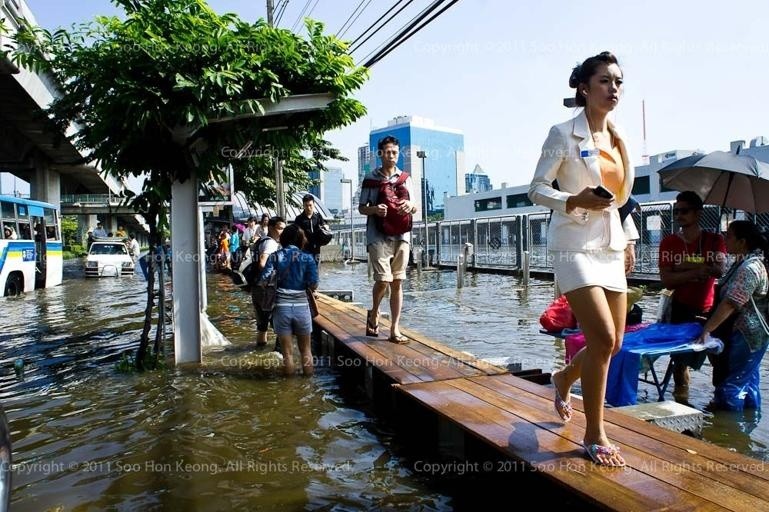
[656,145,769,234]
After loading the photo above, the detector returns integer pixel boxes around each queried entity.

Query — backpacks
[360,170,412,237]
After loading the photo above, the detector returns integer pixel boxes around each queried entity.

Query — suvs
[82,236,136,279]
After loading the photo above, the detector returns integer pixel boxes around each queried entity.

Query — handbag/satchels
[259,270,279,312]
[314,221,333,247]
[656,288,674,322]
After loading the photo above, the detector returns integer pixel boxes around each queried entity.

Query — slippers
[366,310,380,337]
[387,333,410,344]
[550,369,572,423]
[580,440,626,467]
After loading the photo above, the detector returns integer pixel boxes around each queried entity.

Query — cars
[0,404,17,512]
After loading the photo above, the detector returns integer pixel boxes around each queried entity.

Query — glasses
[672,207,691,215]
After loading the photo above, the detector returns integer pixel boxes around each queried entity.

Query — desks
[538,325,719,402]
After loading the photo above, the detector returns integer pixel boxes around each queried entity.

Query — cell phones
[594,185,613,199]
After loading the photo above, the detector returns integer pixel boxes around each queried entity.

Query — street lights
[415,149,433,266]
[338,178,354,264]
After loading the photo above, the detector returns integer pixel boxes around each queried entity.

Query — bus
[0,195,65,301]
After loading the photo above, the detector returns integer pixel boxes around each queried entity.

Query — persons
[697,220,769,410]
[528,52,640,467]
[294,194,326,299]
[261,224,320,373]
[3,215,55,240]
[87,222,172,281]
[358,136,417,344]
[251,216,286,347]
[658,191,727,386]
[211,213,270,272]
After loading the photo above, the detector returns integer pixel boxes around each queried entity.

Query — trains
[327,191,746,274]
